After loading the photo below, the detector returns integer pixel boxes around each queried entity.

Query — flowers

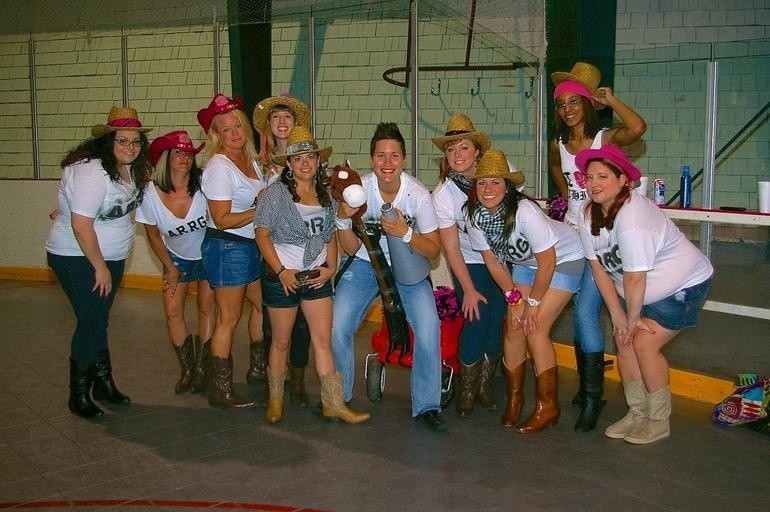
[544,192,570,221]
[432,281,459,321]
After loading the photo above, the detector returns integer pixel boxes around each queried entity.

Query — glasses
[112,136,145,148]
[174,147,196,160]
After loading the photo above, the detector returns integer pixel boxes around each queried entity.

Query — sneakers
[314,398,352,417]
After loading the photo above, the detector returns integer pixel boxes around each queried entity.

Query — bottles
[678,165,692,209]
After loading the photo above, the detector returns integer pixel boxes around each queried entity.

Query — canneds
[655,179,664,205]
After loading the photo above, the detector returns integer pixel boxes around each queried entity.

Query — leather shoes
[418,407,449,432]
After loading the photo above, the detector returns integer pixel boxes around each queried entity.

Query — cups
[635,176,649,197]
[758,181,770,213]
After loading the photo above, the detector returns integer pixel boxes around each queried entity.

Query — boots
[317,373,372,427]
[66,357,105,420]
[264,364,288,423]
[171,334,195,396]
[287,363,310,410]
[259,380,273,408]
[187,333,214,396]
[456,338,673,446]
[248,338,270,386]
[207,354,259,412]
[91,349,131,406]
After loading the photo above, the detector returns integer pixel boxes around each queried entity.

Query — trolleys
[357,220,467,414]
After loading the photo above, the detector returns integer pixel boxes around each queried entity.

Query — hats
[89,105,155,138]
[549,61,611,112]
[430,113,492,156]
[270,125,333,166]
[468,148,526,190]
[146,130,207,167]
[196,92,247,136]
[252,95,313,138]
[572,142,643,185]
